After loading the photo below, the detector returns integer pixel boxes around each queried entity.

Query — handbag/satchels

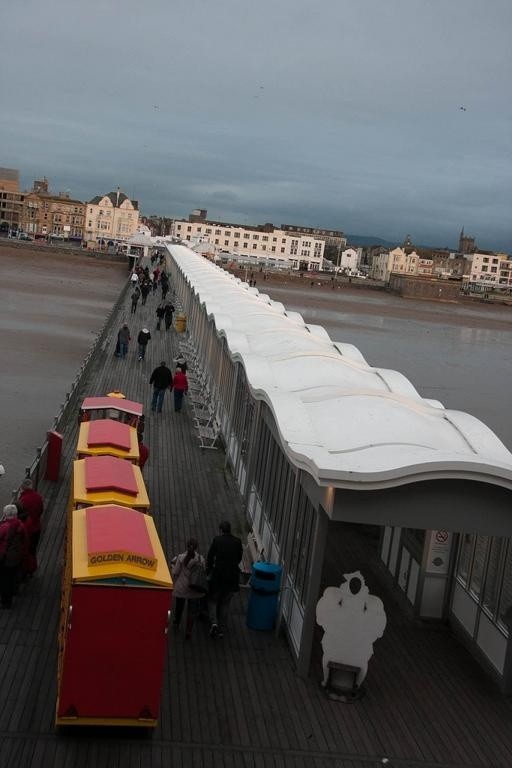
[188,563,208,594]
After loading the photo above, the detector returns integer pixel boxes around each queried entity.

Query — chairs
[173,295,222,450]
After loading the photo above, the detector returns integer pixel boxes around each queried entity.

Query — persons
[237,264,353,290]
[207,520,244,640]
[117,250,190,413]
[170,536,207,640]
[0,478,44,608]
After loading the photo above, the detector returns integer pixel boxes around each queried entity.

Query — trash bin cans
[175,315,186,334]
[248,562,282,631]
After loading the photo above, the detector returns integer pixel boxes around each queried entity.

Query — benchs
[237,521,264,587]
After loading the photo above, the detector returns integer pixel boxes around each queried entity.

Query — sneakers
[209,623,225,636]
[172,619,193,640]
